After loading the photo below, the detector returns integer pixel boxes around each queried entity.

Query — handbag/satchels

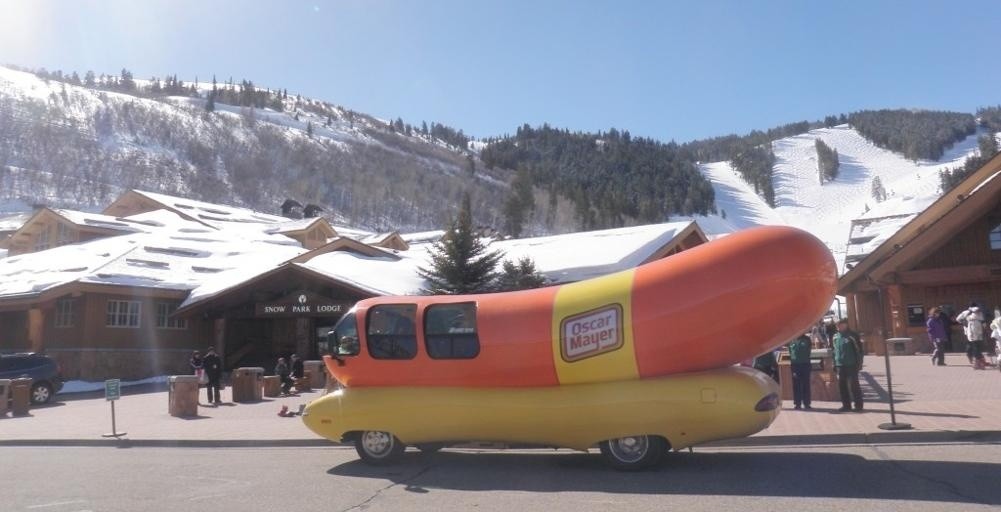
[195,368,209,385]
[218,376,225,389]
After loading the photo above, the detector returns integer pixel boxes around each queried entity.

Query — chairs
[368,332,481,360]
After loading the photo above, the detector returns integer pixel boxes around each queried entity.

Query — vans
[0,352,64,405]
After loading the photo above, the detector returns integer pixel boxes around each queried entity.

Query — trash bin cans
[809,348,836,401]
[1,377,32,417]
[303,360,320,388]
[233,367,264,403]
[168,375,199,416]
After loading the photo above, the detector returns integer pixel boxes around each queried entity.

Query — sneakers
[938,361,946,365]
[795,404,811,409]
[839,407,862,412]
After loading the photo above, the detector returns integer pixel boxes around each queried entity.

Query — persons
[189,350,204,406]
[274,358,294,396]
[751,313,865,413]
[925,301,1001,371]
[203,346,222,406]
[289,354,304,379]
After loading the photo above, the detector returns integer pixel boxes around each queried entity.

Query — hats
[837,318,848,324]
